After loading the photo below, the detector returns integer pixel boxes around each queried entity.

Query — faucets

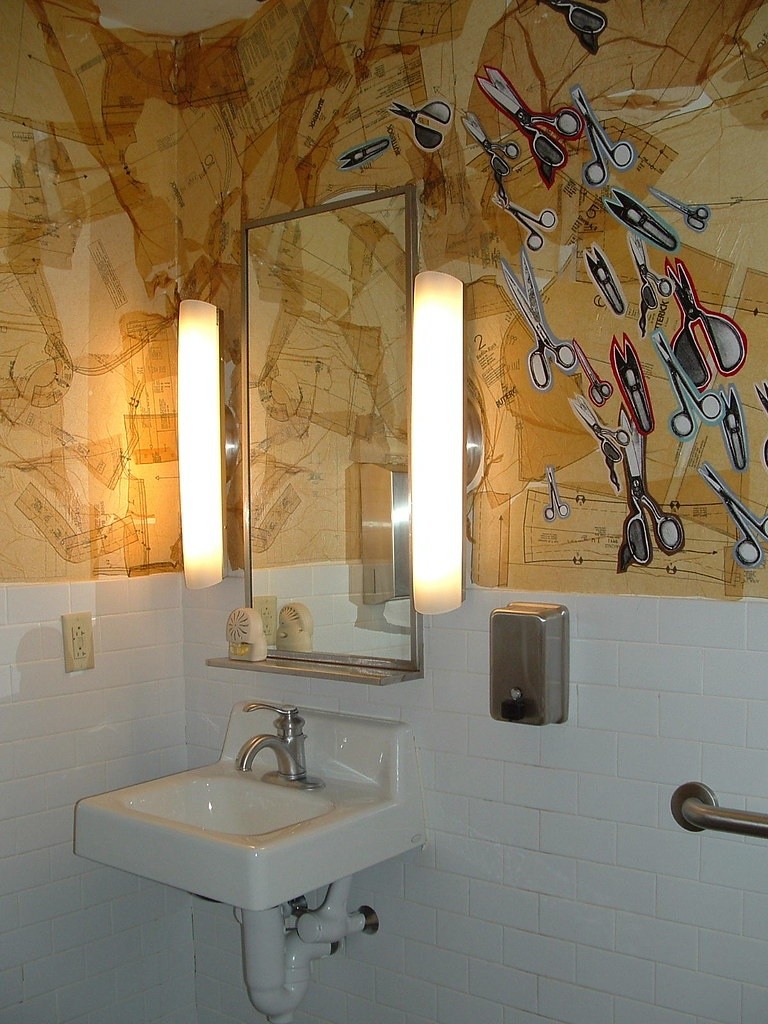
[233,703,326,792]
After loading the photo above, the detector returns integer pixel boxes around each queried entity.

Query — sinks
[74,699,430,917]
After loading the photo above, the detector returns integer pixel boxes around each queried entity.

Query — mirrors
[243,184,424,671]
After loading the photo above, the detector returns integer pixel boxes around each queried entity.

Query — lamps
[178,300,222,590]
[410,270,464,615]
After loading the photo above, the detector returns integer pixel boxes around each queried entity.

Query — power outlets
[253,595,277,645]
[62,612,94,673]
